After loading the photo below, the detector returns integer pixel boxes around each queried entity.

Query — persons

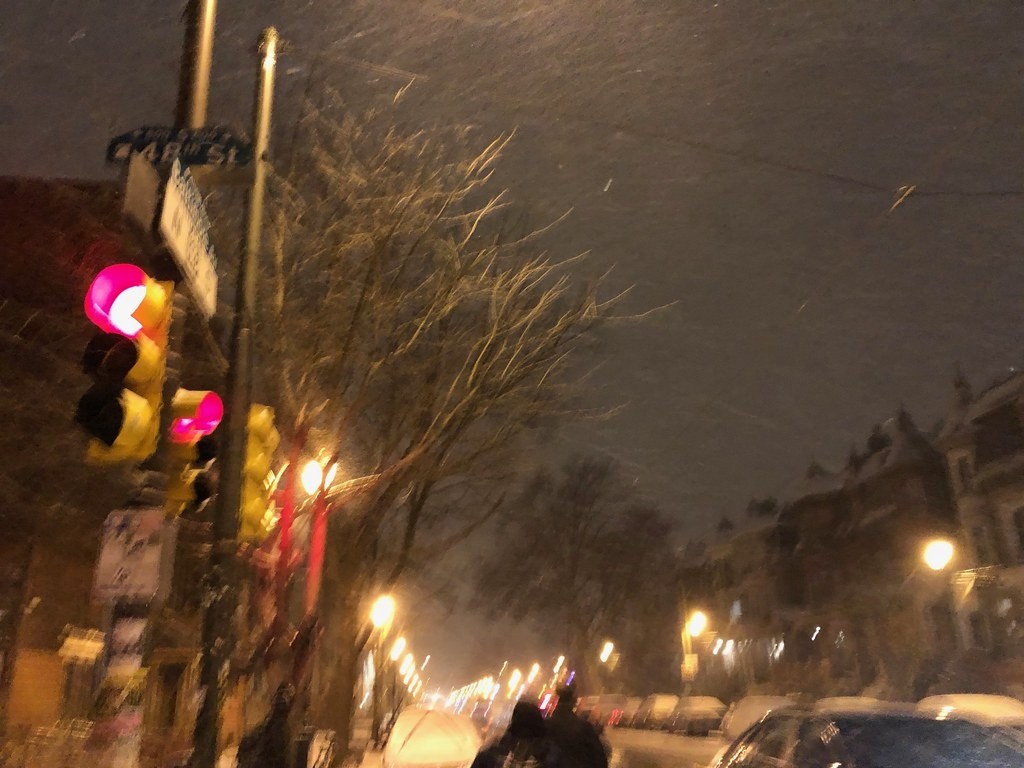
[471,683,609,768]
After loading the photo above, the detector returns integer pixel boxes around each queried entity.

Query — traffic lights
[73,261,177,461]
[151,283,224,478]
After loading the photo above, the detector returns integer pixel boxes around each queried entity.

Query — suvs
[705,703,1024,768]
[577,690,1024,739]
